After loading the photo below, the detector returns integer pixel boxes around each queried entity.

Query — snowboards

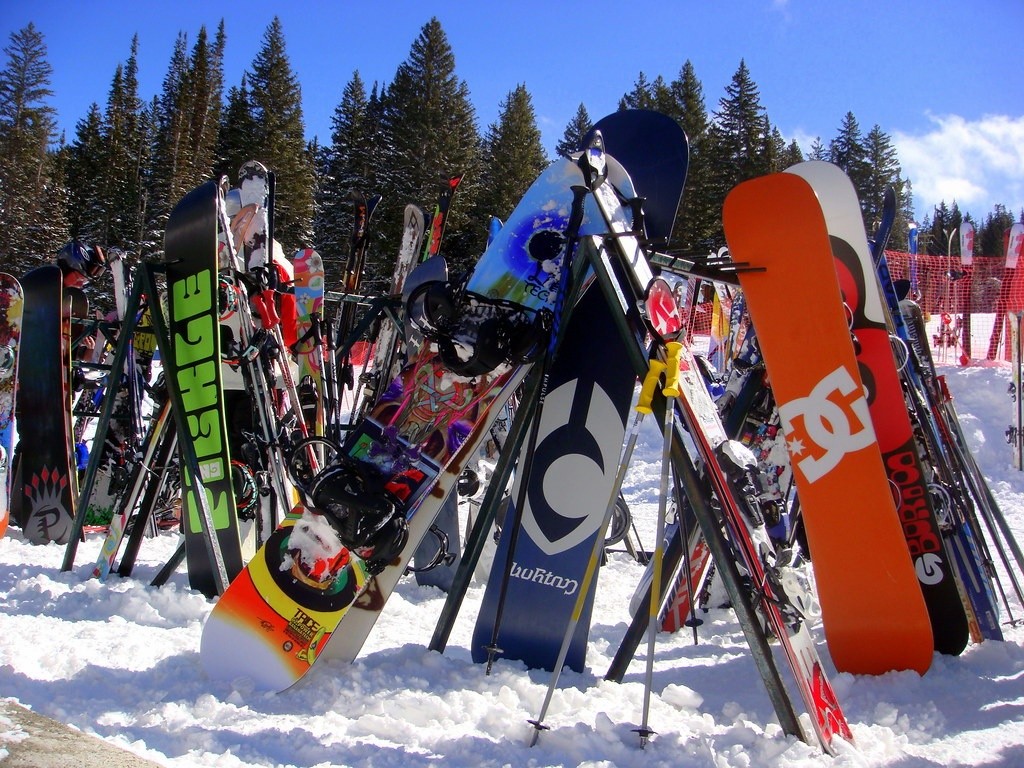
[0,106,1023,758]
